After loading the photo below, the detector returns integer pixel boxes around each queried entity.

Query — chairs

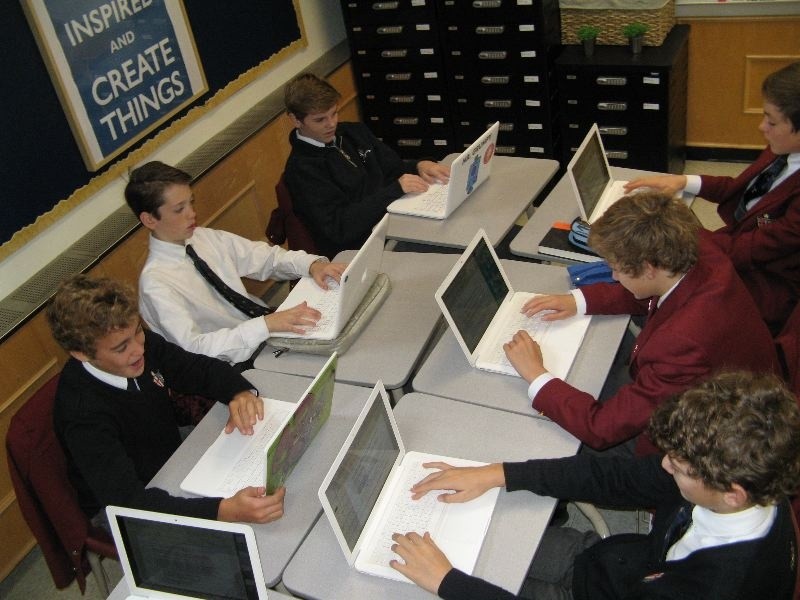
[265,172,320,291]
[8,374,120,599]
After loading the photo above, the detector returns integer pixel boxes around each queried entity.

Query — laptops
[386,120,500,221]
[180,350,339,497]
[105,504,268,600]
[433,227,666,383]
[318,379,502,586]
[566,122,684,225]
[266,211,390,340]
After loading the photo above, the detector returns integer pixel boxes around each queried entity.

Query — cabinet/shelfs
[340,0,687,175]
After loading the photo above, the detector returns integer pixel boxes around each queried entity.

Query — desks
[107,152,695,600]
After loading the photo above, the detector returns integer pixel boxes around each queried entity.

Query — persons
[502,190,788,454]
[124,161,349,368]
[622,58,799,336]
[279,73,451,237]
[25,276,286,540]
[388,369,800,600]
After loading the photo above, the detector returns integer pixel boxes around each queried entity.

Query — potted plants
[623,23,649,54]
[575,25,601,58]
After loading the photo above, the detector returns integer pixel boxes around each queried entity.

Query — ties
[186,244,274,318]
[656,506,692,561]
[734,153,788,221]
